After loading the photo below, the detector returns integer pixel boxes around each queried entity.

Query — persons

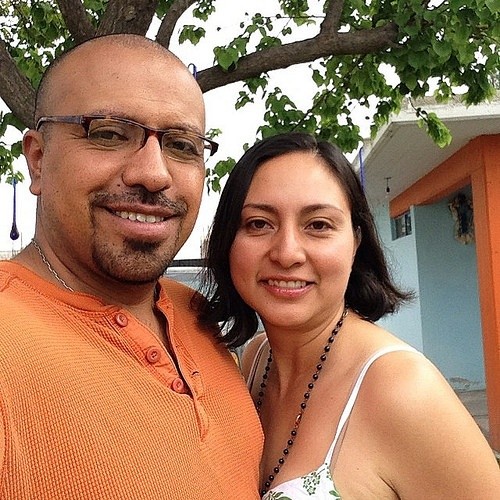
[193,133,499,500]
[0,34,264,500]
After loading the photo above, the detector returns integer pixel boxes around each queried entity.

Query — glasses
[36,115,219,165]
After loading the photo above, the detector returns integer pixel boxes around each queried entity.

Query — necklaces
[255,302,350,498]
[32,238,75,293]
